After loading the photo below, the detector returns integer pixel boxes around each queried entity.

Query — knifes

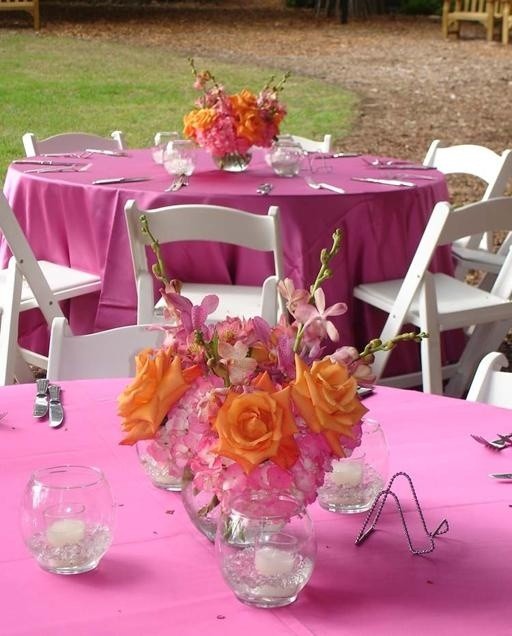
[349,176,403,186]
[491,473,512,479]
[266,136,295,168]
[33,378,50,420]
[47,385,65,428]
[92,176,156,186]
[364,178,416,187]
[86,148,128,157]
[11,158,90,166]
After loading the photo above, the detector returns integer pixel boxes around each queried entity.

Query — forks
[39,151,87,156]
[172,175,189,193]
[302,175,345,195]
[497,432,512,444]
[164,176,179,192]
[470,433,512,451]
[23,162,94,173]
[360,156,411,167]
[45,152,95,159]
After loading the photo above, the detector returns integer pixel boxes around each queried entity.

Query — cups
[315,419,392,517]
[268,142,303,177]
[20,464,113,579]
[215,494,317,610]
[137,438,193,493]
[154,131,182,165]
[161,141,194,177]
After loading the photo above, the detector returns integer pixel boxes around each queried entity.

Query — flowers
[119,214,435,522]
[183,57,291,157]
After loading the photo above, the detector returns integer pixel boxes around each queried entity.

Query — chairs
[21,130,130,157]
[467,350,512,410]
[0,1,41,30]
[422,139,512,249]
[123,199,290,328]
[44,318,176,382]
[353,195,512,394]
[1,188,100,385]
[494,1,512,45]
[449,233,512,294]
[442,0,494,43]
[276,134,334,153]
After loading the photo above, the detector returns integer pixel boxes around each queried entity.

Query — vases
[212,146,252,173]
[182,476,231,544]
